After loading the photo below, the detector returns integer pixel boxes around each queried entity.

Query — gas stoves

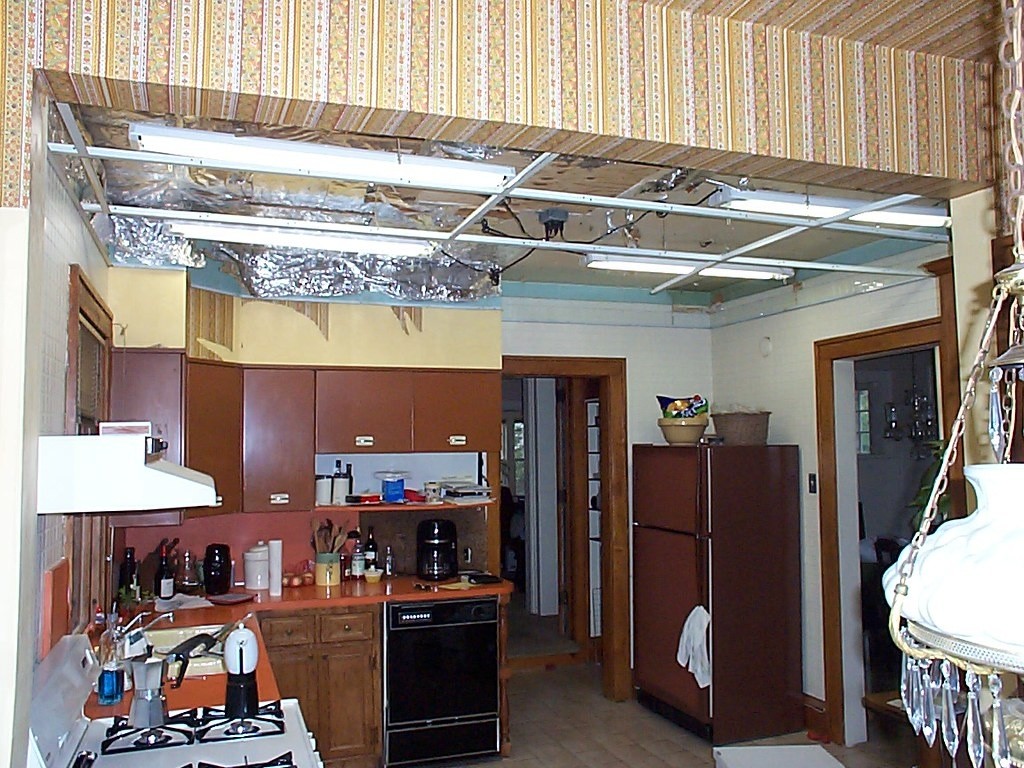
[24,634,323,768]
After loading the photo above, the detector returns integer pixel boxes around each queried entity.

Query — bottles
[384,546,394,576]
[243,541,268,590]
[333,460,353,494]
[98,602,124,705]
[364,525,378,570]
[180,549,194,588]
[335,526,365,581]
[154,545,175,600]
[118,547,136,595]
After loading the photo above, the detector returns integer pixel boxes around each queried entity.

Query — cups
[425,481,441,502]
[315,553,340,586]
[382,478,405,502]
[203,543,232,596]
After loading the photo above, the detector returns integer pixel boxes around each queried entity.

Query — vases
[657,418,708,447]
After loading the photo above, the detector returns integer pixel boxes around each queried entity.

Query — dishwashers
[379,594,503,768]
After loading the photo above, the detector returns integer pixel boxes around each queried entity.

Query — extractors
[36,434,222,514]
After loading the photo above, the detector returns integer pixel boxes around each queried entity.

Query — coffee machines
[415,517,459,583]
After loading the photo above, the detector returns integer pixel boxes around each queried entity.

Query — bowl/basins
[361,569,384,583]
[658,418,709,446]
[710,411,771,445]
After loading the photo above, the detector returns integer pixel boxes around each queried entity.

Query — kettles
[118,644,189,728]
[223,622,259,718]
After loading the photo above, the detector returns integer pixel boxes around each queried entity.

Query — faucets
[116,609,175,639]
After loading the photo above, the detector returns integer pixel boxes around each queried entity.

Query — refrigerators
[631,443,806,747]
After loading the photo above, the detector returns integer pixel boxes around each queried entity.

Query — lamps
[710,188,949,229]
[883,0,1023,768]
[162,219,430,258]
[585,252,795,279]
[127,123,516,191]
[883,353,936,460]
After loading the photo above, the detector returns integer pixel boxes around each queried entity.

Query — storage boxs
[712,746,845,768]
[383,479,404,502]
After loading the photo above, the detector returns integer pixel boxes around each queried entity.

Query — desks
[862,691,972,768]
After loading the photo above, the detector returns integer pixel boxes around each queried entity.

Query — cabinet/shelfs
[108,347,244,526]
[316,367,502,452]
[584,398,603,637]
[244,366,315,510]
[254,603,384,768]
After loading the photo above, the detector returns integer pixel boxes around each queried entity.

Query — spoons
[310,517,321,553]
[332,534,346,553]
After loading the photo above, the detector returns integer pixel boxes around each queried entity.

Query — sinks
[131,624,228,676]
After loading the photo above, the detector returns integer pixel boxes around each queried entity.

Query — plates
[206,593,258,604]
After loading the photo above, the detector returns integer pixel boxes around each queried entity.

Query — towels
[675,605,712,688]
